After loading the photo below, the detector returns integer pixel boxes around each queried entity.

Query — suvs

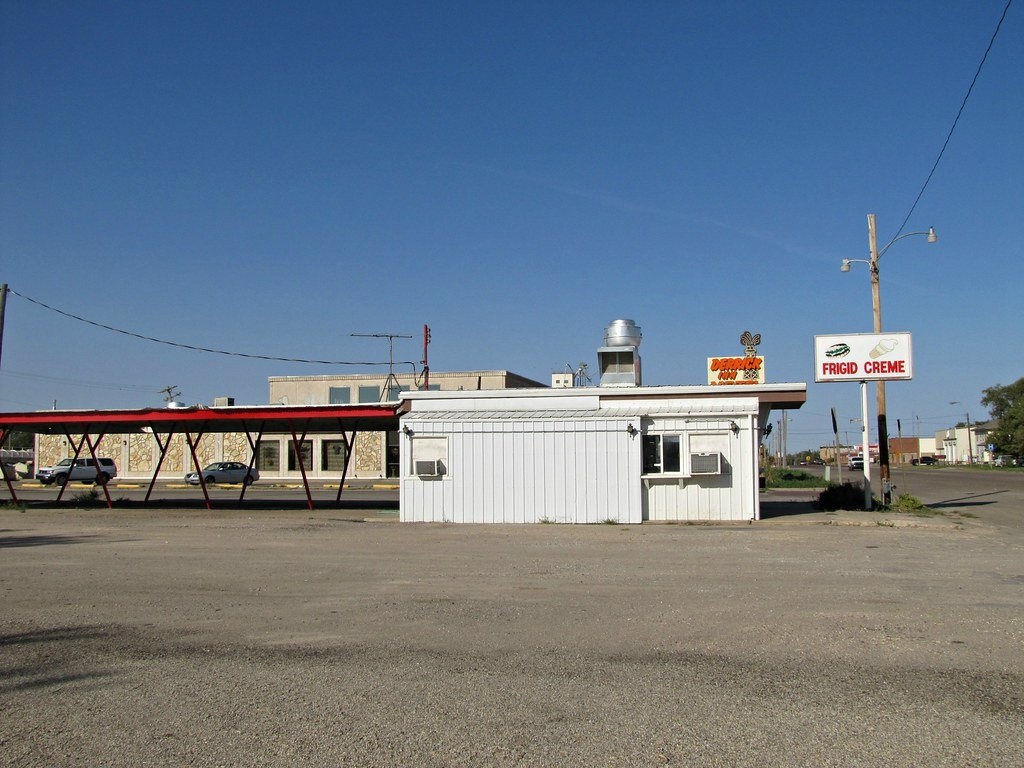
[35,457,117,486]
[847,456,864,471]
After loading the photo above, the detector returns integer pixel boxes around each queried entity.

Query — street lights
[951,401,973,467]
[840,213,937,507]
[779,418,792,466]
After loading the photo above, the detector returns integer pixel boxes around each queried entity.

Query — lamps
[731,421,738,431]
[628,424,633,433]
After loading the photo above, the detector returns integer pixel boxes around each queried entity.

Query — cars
[813,458,825,465]
[800,460,807,466]
[911,456,938,467]
[184,461,260,486]
